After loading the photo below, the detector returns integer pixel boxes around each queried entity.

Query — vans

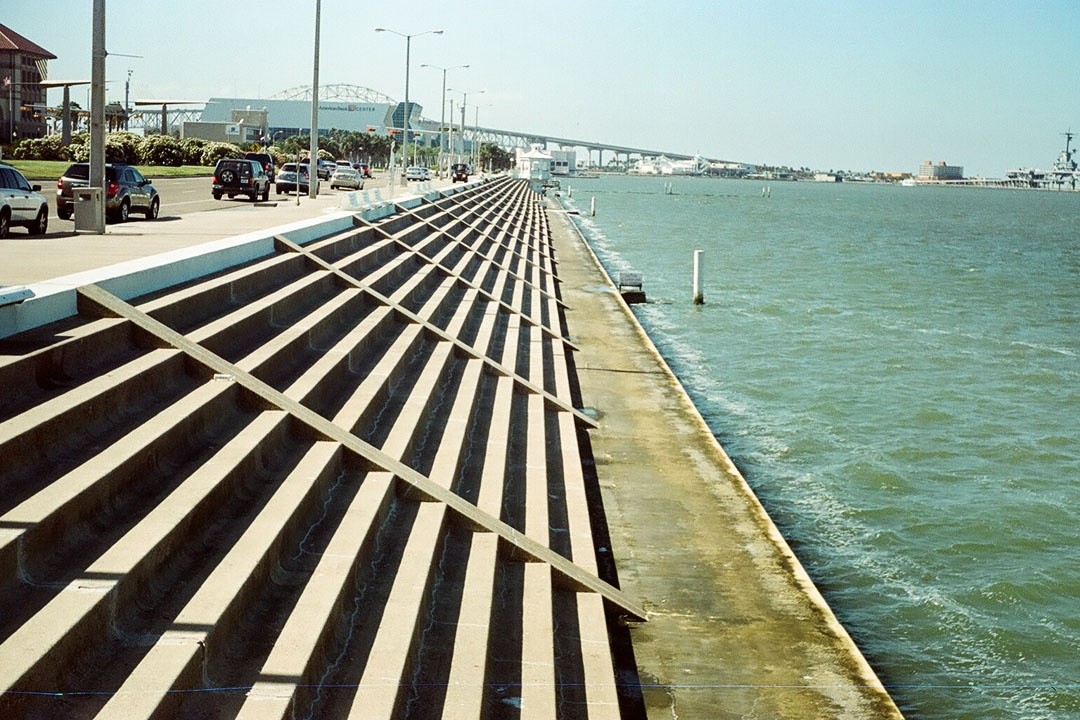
[245,152,275,183]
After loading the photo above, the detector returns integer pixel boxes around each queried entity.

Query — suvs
[0,159,48,238]
[211,158,270,202]
[56,159,160,223]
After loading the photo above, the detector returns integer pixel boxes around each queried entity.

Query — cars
[300,157,372,181]
[276,163,321,196]
[452,170,468,183]
[446,163,473,176]
[420,168,432,180]
[406,167,424,182]
[330,168,364,190]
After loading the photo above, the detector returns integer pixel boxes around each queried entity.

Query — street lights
[445,87,486,164]
[419,64,471,181]
[375,27,444,187]
[463,103,492,171]
